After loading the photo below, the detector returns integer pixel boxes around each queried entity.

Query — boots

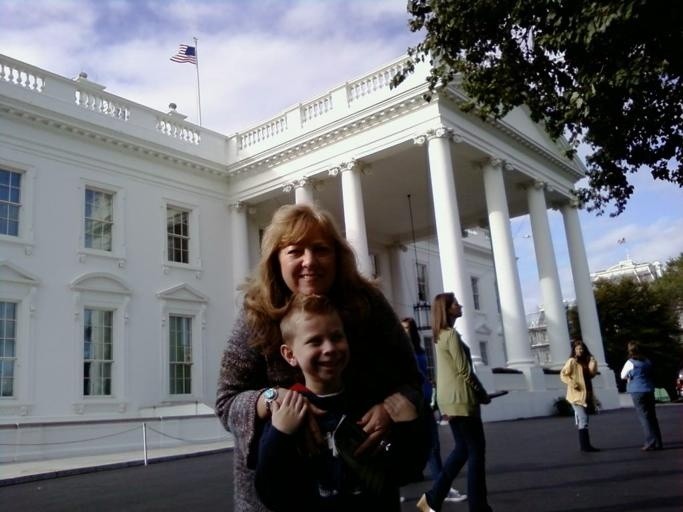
[579,429,600,452]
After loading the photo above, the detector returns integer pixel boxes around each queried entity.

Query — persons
[397,314,468,505]
[254,290,434,510]
[557,339,604,456]
[213,198,437,511]
[617,335,664,453]
[411,291,494,512]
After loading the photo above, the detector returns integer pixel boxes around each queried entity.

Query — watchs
[263,383,279,417]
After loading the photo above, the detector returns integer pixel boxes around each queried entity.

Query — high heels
[416,493,435,512]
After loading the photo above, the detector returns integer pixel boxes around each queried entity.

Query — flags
[168,45,197,66]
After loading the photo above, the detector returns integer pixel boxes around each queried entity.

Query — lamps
[406,194,432,330]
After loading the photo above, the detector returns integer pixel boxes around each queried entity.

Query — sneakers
[641,445,662,451]
[445,488,467,502]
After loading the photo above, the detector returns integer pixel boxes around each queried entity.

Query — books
[488,390,507,399]
